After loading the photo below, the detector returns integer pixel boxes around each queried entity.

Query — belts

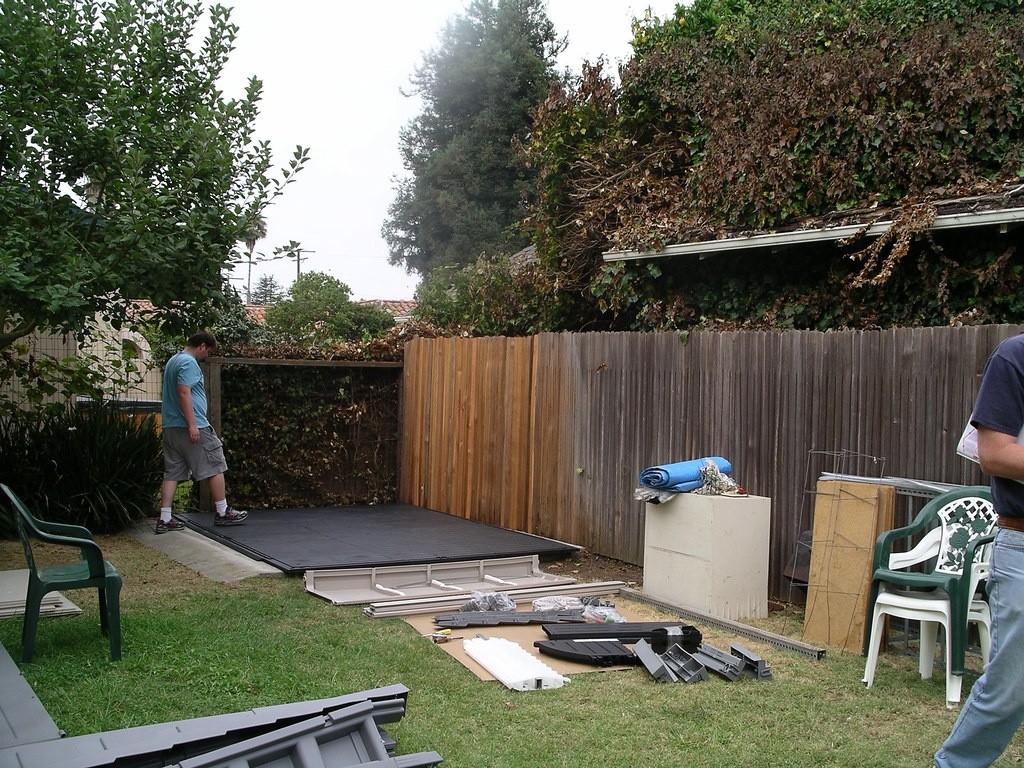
[998,515,1024,532]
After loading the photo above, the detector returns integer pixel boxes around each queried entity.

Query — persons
[156,332,248,532]
[933,335,1024,768]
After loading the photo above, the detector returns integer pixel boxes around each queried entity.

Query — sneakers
[214,506,248,525]
[156,519,185,533]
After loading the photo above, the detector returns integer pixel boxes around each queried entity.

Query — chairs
[0,483,123,664]
[860,485,1000,710]
